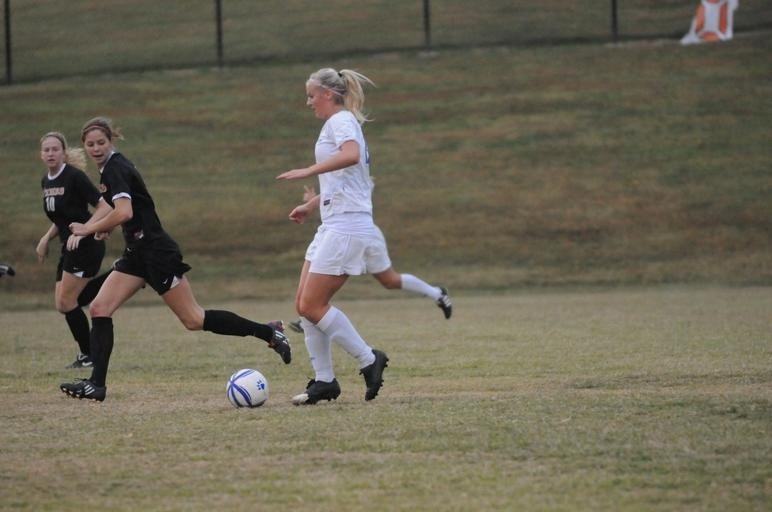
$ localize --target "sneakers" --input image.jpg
[360,349,390,401]
[437,287,452,320]
[61,378,107,403]
[0,264,15,277]
[267,320,293,365]
[65,353,94,370]
[288,320,305,334]
[292,378,342,407]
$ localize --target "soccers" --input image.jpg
[226,370,269,407]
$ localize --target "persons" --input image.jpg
[60,117,291,402]
[276,68,389,406]
[36,132,145,369]
[289,184,452,334]
